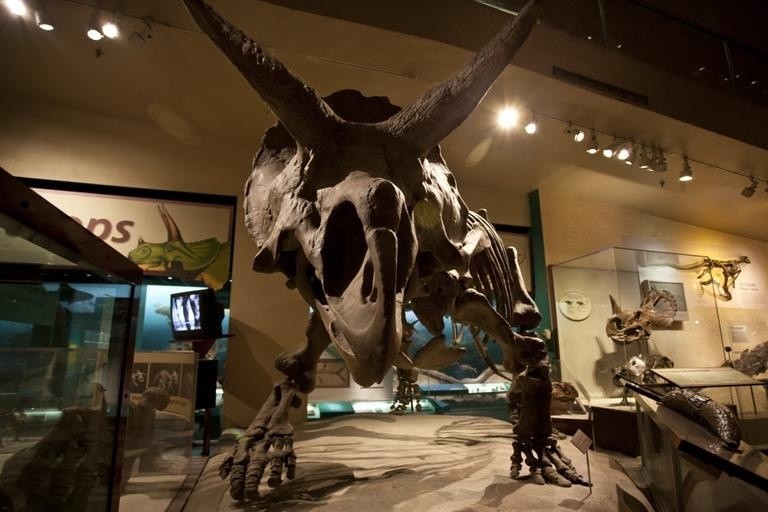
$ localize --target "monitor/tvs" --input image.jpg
[170,288,222,340]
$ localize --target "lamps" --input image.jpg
[740,178,760,198]
[563,123,694,184]
[34,3,155,54]
